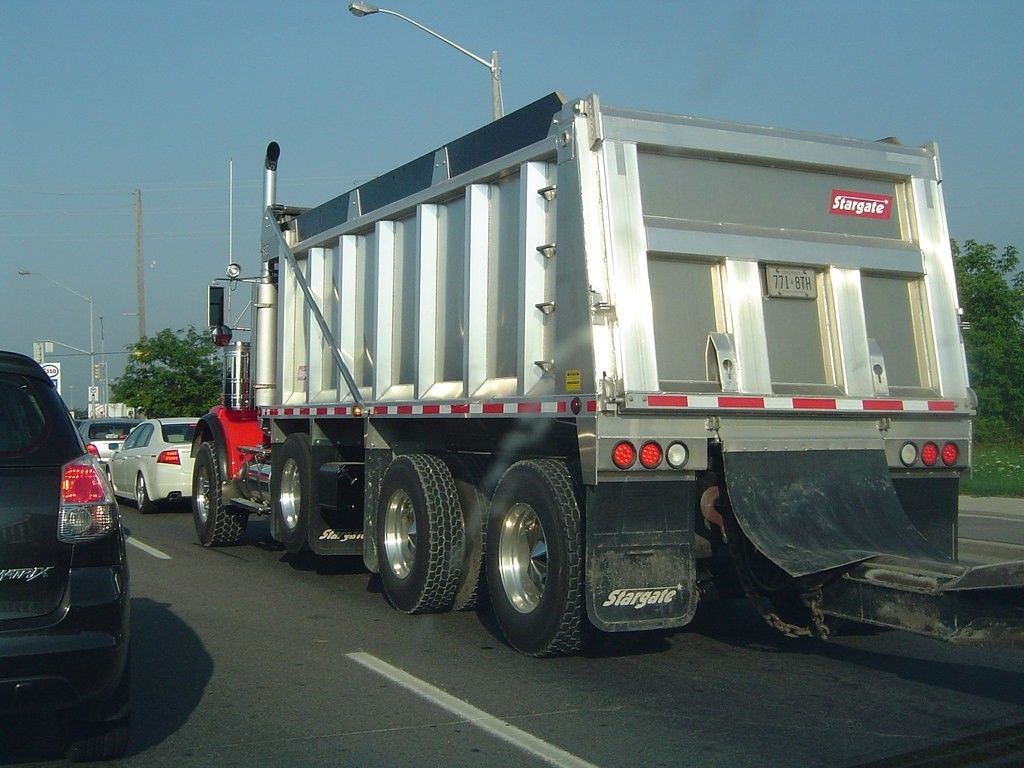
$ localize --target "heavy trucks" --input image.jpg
[190,91,1024,658]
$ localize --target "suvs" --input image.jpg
[0,351,130,762]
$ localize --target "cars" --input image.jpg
[74,418,141,462]
[106,417,205,515]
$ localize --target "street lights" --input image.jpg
[19,269,95,420]
